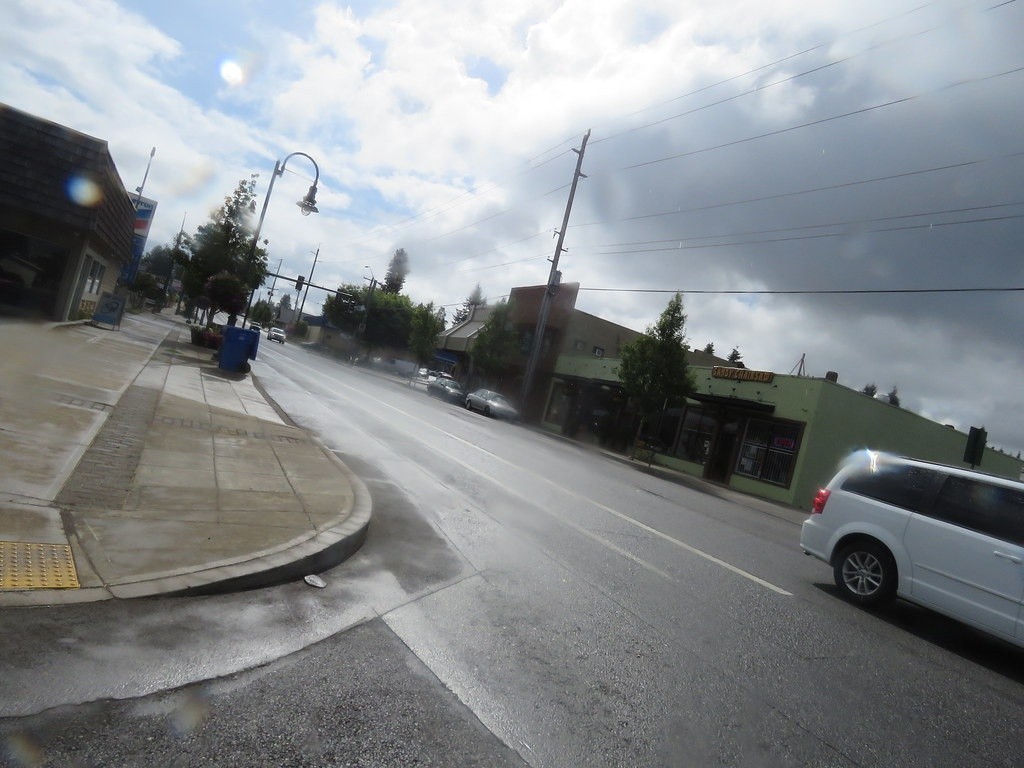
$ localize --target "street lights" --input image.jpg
[212,153,319,359]
[349,265,377,363]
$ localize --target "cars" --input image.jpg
[427,379,464,403]
[384,359,419,377]
[249,322,260,331]
[429,370,441,376]
[800,448,1024,651]
[464,389,518,418]
[267,327,286,344]
[419,368,428,376]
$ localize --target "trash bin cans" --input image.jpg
[218,325,260,374]
